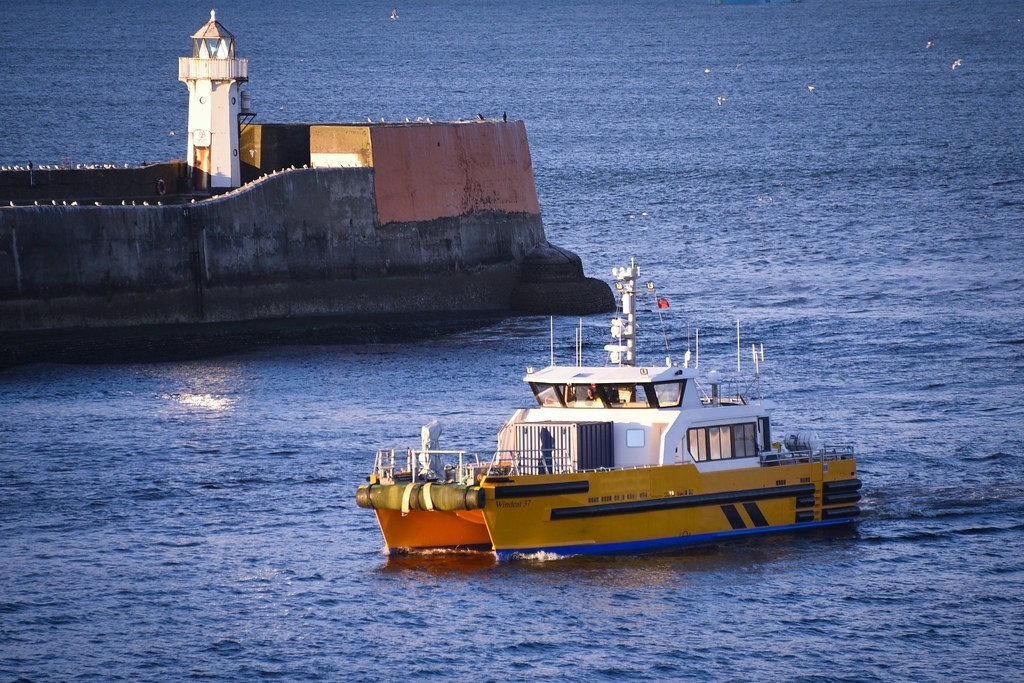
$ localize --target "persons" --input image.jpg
[586,388,597,401]
[502,112,507,122]
[142,160,146,165]
[29,161,33,171]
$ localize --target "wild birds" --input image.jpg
[689,35,963,107]
[2,106,512,217]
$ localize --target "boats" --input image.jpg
[354,252,862,560]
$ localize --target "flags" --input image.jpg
[657,298,670,308]
